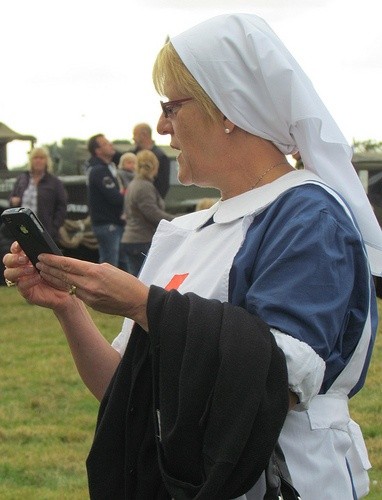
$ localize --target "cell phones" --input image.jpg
[2,208,63,275]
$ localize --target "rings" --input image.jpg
[69,285,77,294]
[5,279,20,287]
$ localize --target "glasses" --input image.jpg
[160,97,192,118]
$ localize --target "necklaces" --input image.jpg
[248,160,289,190]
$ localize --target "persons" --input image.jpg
[10,145,68,244]
[86,122,180,279]
[3,12,382,499]
